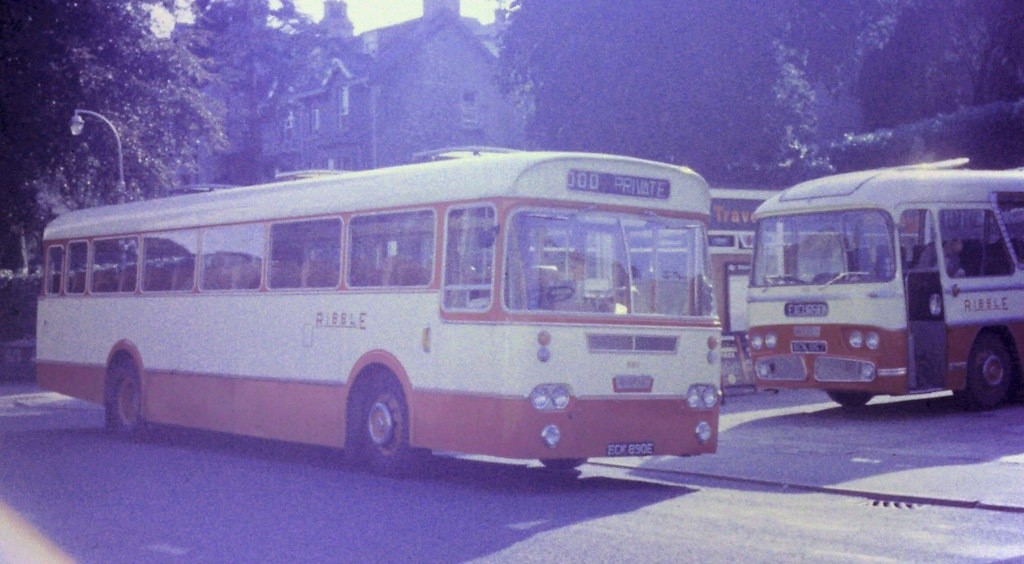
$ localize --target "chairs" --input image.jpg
[52,259,428,286]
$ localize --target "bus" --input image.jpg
[541,184,922,392]
[748,155,1024,411]
[35,145,722,478]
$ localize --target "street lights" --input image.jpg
[71,106,125,209]
